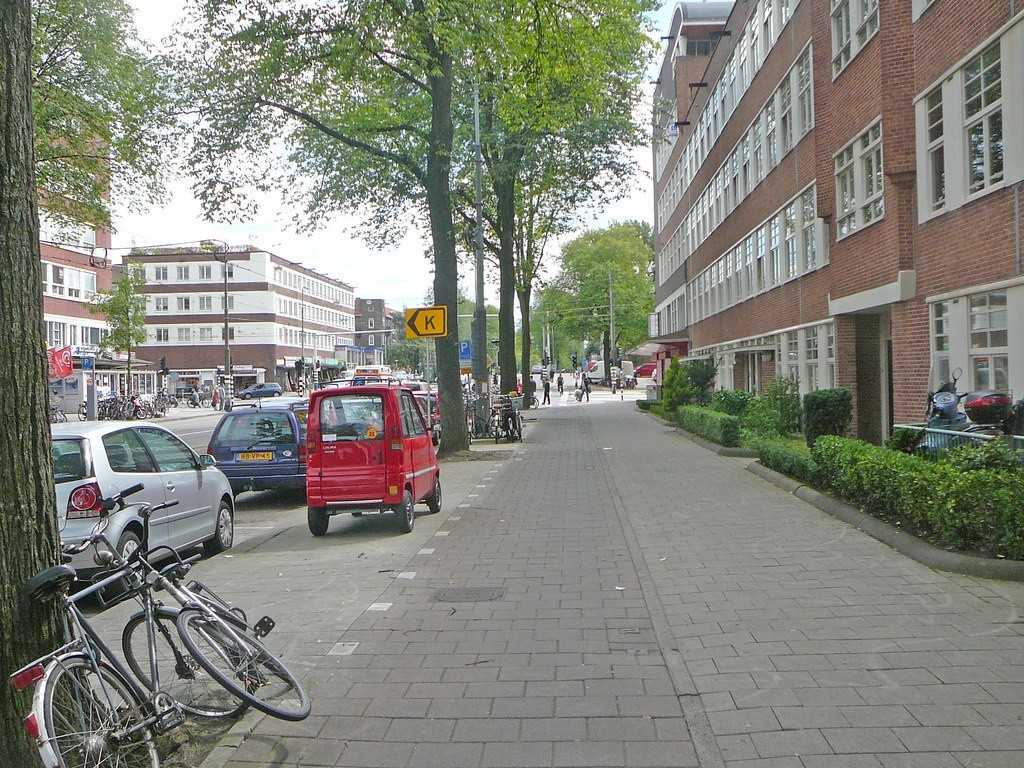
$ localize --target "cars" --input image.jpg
[237,382,283,399]
[206,408,306,502]
[52,419,234,600]
[318,363,446,420]
[304,385,445,535]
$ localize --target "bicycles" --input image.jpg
[186,390,212,408]
[50,381,179,422]
[557,379,564,395]
[93,498,312,722]
[8,483,257,768]
[462,382,523,444]
[529,397,540,409]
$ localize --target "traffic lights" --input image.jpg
[572,351,577,369]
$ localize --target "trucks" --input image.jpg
[585,360,634,384]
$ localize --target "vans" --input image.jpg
[633,363,657,377]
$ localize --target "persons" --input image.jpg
[517,379,522,389]
[556,374,564,395]
[530,375,537,396]
[578,372,590,402]
[550,368,555,383]
[212,389,220,405]
[190,389,201,407]
[541,379,551,405]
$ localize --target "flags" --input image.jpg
[51,346,74,379]
[45,348,59,380]
